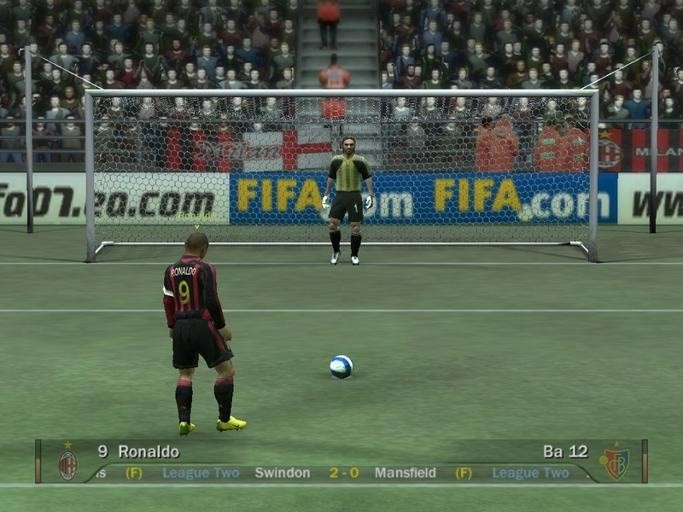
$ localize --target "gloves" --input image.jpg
[365,194,374,210]
[322,195,330,210]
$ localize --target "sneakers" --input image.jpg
[330,251,340,266]
[351,254,360,267]
[178,421,197,438]
[216,415,247,433]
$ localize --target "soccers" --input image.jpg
[330,355,353,378]
